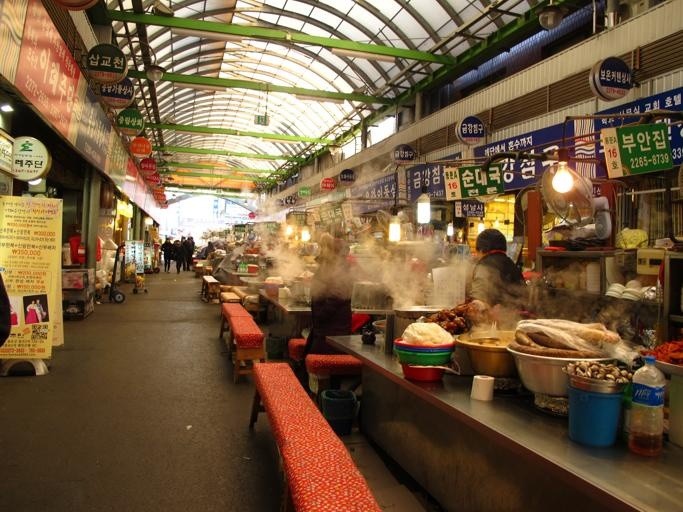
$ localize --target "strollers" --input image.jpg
[132,272,148,294]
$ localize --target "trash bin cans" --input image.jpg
[267,335,283,359]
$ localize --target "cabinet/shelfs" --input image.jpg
[536,249,682,373]
[62,266,96,319]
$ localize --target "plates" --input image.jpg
[604,279,657,301]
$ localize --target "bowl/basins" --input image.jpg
[240,248,260,274]
[394,304,455,320]
[398,360,453,382]
[287,275,312,306]
[561,366,632,395]
[456,329,518,377]
[263,276,285,298]
[393,337,457,365]
[372,319,385,336]
[505,342,619,396]
[586,261,600,294]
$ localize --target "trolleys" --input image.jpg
[99,243,127,304]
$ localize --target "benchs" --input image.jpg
[306,354,361,395]
[248,363,382,512]
[195,262,266,386]
[288,338,308,361]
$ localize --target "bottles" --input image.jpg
[630,356,666,459]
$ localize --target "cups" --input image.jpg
[362,326,376,344]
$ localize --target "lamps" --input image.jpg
[302,206,311,242]
[551,116,576,193]
[417,161,431,226]
[447,210,502,236]
[121,41,165,82]
[388,162,402,241]
[539,0,562,29]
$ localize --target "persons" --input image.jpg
[465,228,529,308]
[161,236,196,274]
[305,231,352,354]
[25,299,39,323]
[203,242,215,259]
[35,299,46,321]
